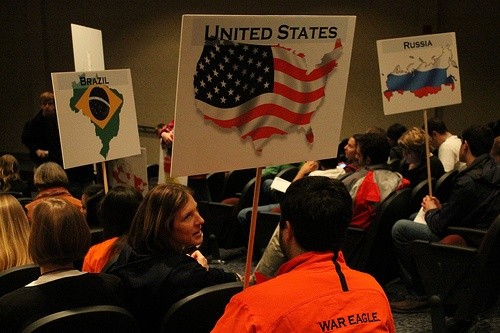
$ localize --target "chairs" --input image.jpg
[0,264,40,297]
[23,306,133,333]
[412,214,500,333]
[165,282,244,333]
[198,170,458,260]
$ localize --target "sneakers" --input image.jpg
[389,292,436,315]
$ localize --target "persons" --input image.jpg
[208,176,396,333]
[388,123,499,313]
[102,184,242,333]
[0,92,468,272]
[0,197,131,333]
